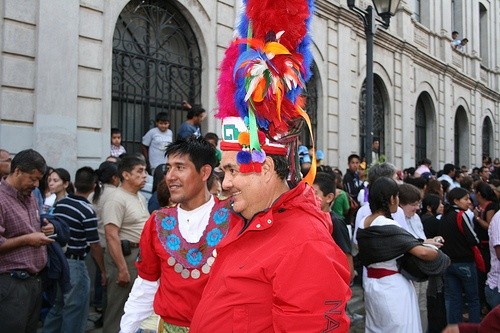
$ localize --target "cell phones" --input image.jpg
[47,233,58,239]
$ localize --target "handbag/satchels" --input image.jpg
[473,245,486,273]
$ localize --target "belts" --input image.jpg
[65,253,85,260]
[365,266,395,280]
[129,242,139,248]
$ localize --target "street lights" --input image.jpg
[346,0,402,171]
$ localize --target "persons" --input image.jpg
[0,148,178,333]
[451,31,459,40]
[452,38,469,51]
[204,131,222,165]
[176,99,206,140]
[117,134,245,333]
[342,137,500,333]
[294,137,354,254]
[141,112,173,175]
[110,126,126,157]
[189,0,352,333]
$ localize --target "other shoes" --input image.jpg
[93,302,104,312]
